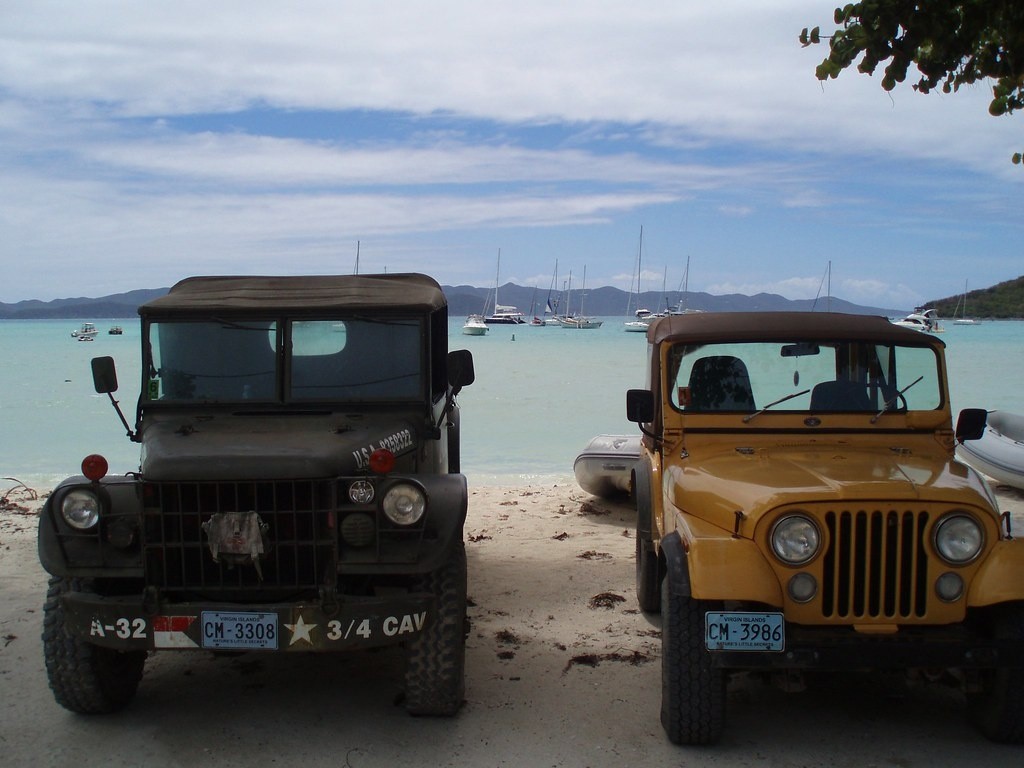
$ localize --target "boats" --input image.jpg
[71,321,123,342]
[953,409,1023,490]
[574,434,649,509]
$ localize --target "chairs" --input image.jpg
[683,355,757,410]
[810,380,875,411]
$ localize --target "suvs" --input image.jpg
[625,310,1023,747]
[36,271,475,718]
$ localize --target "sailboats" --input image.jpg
[622,224,709,332]
[889,276,984,336]
[462,246,605,335]
